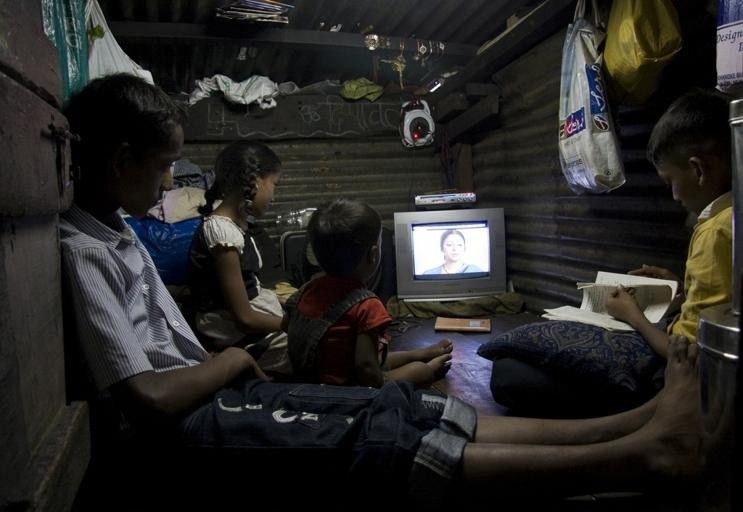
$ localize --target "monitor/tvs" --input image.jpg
[392,207,508,303]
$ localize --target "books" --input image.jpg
[214,0,295,25]
[435,315,490,334]
[541,269,682,332]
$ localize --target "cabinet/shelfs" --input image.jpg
[0,0,93,511]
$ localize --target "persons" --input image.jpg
[489,100,735,420]
[282,199,453,389]
[186,140,291,368]
[423,230,482,273]
[61,72,702,511]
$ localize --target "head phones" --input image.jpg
[397,100,437,150]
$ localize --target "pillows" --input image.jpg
[474,318,664,394]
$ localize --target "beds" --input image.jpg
[375,308,694,512]
